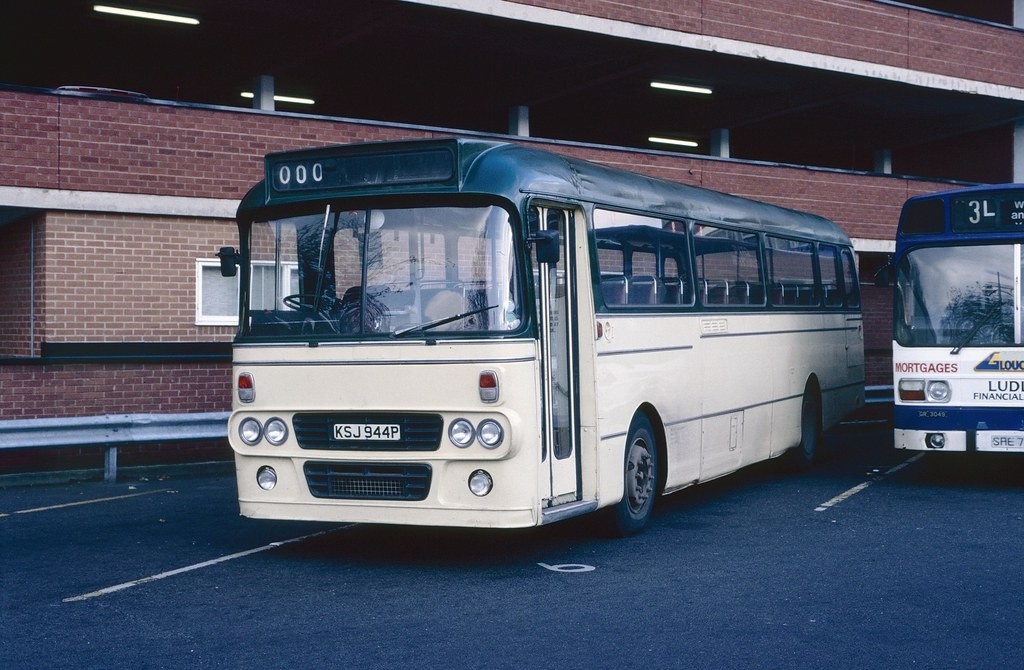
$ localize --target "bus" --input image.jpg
[892,183,1024,451]
[216,138,864,537]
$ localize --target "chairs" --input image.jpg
[334,276,838,332]
